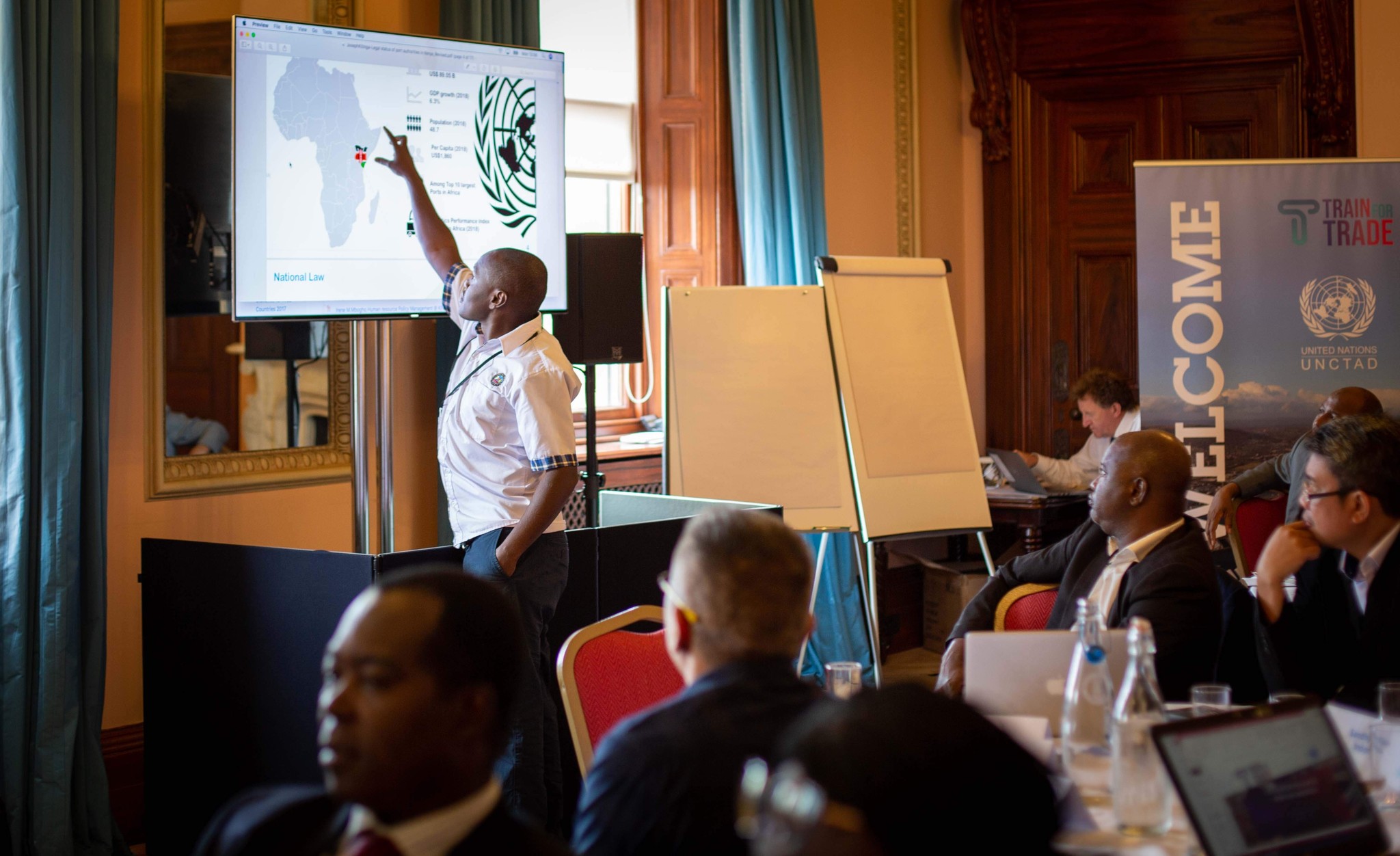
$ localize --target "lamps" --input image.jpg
[548,233,643,526]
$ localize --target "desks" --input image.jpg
[945,487,1097,558]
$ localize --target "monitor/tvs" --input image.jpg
[160,70,232,317]
[230,13,565,320]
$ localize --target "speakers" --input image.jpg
[245,319,327,359]
[552,233,645,365]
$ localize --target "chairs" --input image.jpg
[995,581,1060,634]
[1224,480,1304,585]
[558,597,704,778]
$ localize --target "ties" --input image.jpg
[340,829,403,856]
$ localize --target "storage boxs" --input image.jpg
[891,548,1000,654]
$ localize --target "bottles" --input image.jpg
[1107,617,1177,838]
[1060,599,1118,790]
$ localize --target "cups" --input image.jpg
[1378,682,1400,725]
[1189,685,1231,719]
[1365,722,1399,813]
[825,661,862,701]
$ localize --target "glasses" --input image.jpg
[739,760,860,840]
[1298,487,1373,503]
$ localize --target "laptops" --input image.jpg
[1150,697,1400,856]
[964,632,1135,740]
[983,446,1093,496]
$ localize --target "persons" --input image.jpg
[165,401,229,459]
[196,566,576,856]
[933,369,1400,718]
[574,507,1075,856]
[375,125,582,844]
